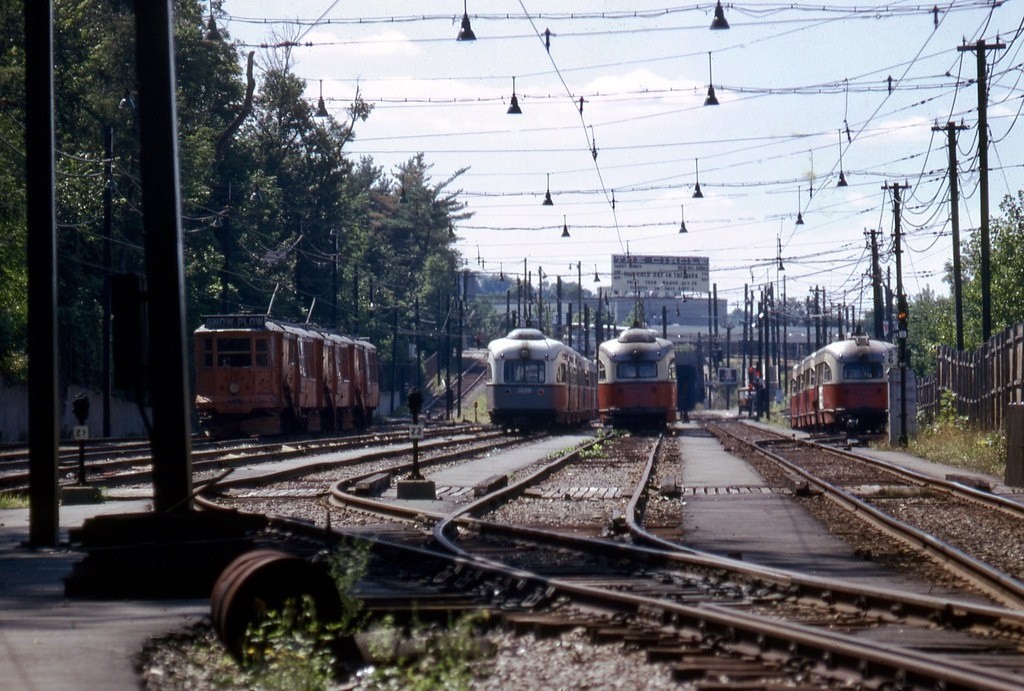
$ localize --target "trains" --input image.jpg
[194,311,380,436]
[789,331,897,434]
[486,327,599,432]
[594,329,679,425]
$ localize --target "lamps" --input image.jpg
[691,158,703,198]
[675,301,680,316]
[593,263,600,282]
[506,77,522,114]
[704,52,719,105]
[679,205,687,233]
[561,215,570,237]
[313,80,329,117]
[795,186,804,224]
[710,0,730,29]
[682,264,688,279]
[456,0,476,41]
[543,173,554,205]
[836,129,848,186]
[498,263,504,282]
[777,239,784,270]
[682,290,686,301]
